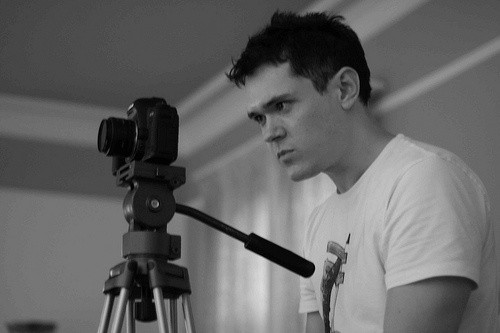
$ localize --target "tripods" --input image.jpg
[97,162,196,333]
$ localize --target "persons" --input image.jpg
[223,6,500,332]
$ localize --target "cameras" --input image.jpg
[98,97,178,175]
